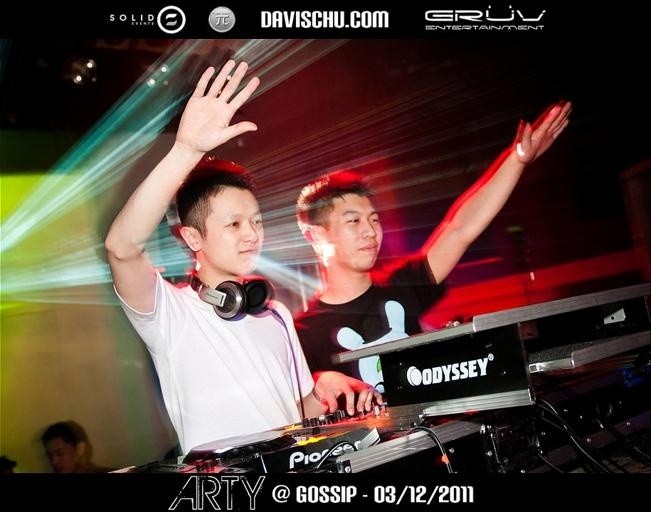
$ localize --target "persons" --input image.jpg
[32,418,119,477]
[285,96,575,404]
[97,55,389,454]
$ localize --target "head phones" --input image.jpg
[191,275,275,321]
